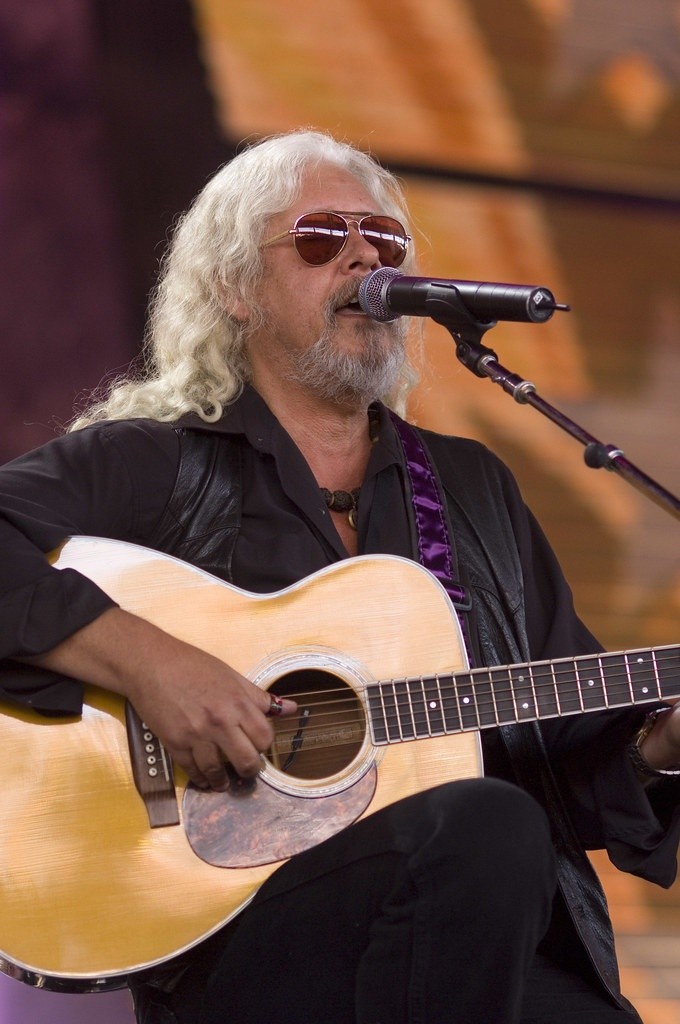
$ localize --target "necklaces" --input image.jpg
[321,486,363,529]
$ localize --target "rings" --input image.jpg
[265,693,282,716]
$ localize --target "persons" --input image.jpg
[0,132,680,1024]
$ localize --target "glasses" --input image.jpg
[257,211,412,269]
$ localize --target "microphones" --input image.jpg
[358,266,571,324]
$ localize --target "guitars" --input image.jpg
[3,529,680,999]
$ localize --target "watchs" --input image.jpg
[624,703,680,779]
